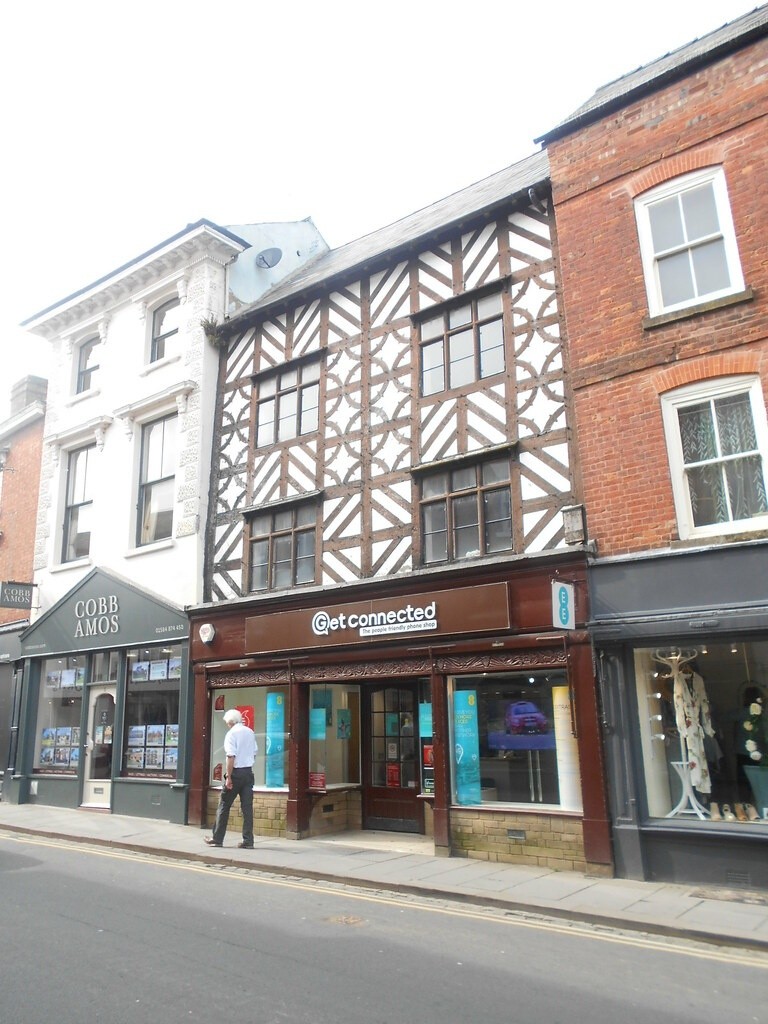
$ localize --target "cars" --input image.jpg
[505,701,548,736]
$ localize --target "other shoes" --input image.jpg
[710,802,722,820]
[746,803,760,821]
[238,843,253,848]
[723,805,735,819]
[735,804,747,820]
[204,835,222,847]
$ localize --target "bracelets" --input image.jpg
[226,774,229,777]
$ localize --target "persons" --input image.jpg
[204,709,257,849]
